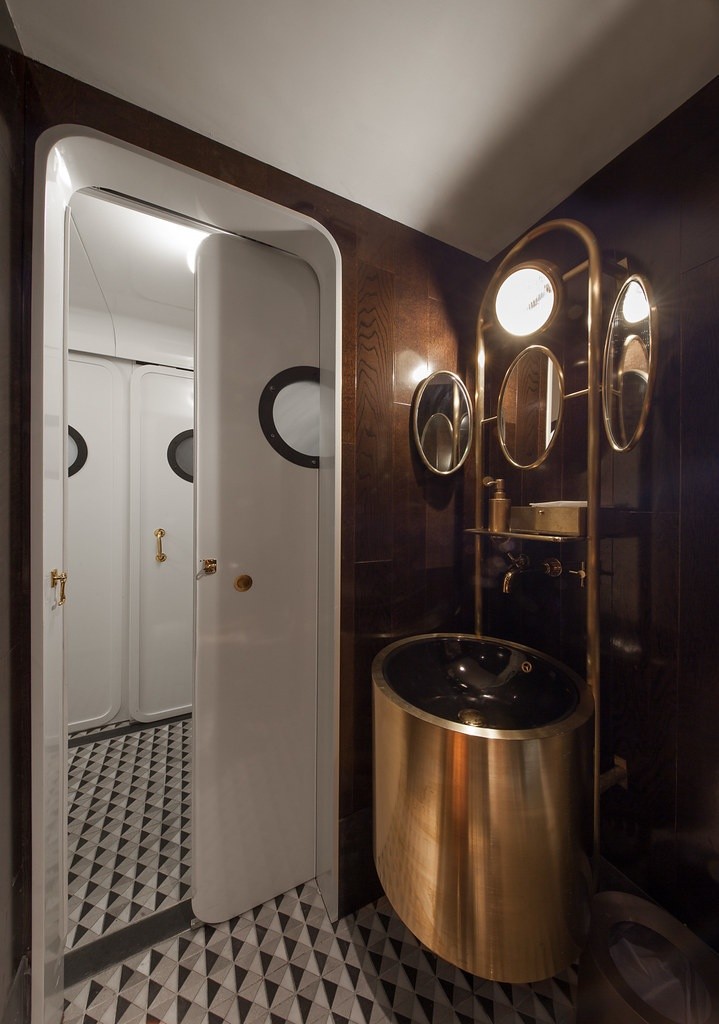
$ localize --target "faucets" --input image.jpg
[499,550,534,598]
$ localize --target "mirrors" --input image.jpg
[498,345,565,471]
[612,334,649,450]
[601,274,658,455]
[422,414,470,473]
[413,371,474,476]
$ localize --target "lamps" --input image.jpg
[492,258,566,341]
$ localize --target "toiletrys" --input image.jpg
[483,478,511,534]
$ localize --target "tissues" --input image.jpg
[508,499,628,540]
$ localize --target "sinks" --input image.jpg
[366,629,594,987]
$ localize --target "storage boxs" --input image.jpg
[511,505,617,537]
